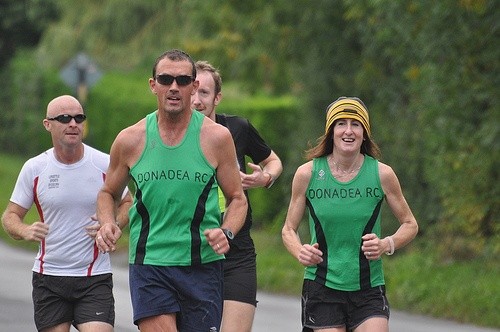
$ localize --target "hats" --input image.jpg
[326,97,370,138]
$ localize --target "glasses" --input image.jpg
[153,74,195,86]
[47,115,87,124]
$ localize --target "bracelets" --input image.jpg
[384,237,394,256]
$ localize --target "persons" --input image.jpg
[189,59,282,332]
[1,95,134,332]
[281,96,418,332]
[96,48,248,332]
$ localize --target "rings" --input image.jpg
[217,244,221,249]
[368,252,371,256]
[97,236,102,239]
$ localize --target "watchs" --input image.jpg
[265,172,275,189]
[219,227,234,246]
